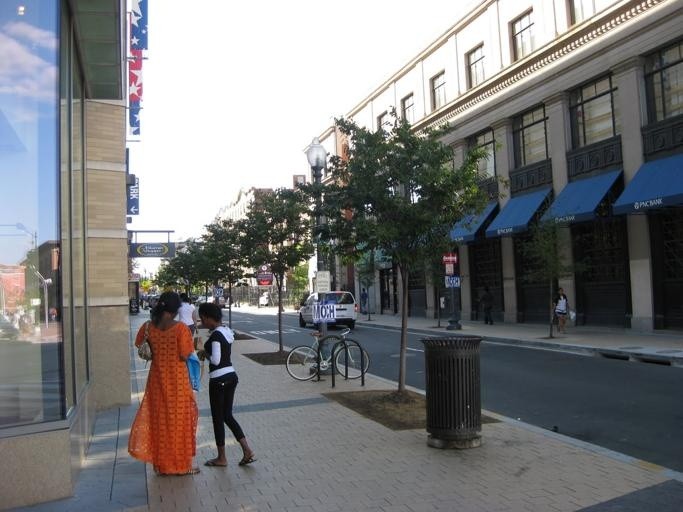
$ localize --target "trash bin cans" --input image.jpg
[416,335,485,449]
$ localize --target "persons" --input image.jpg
[4,310,34,339]
[177,292,198,353]
[360,288,368,314]
[140,295,159,308]
[480,286,494,324]
[188,299,196,310]
[552,288,570,334]
[127,291,201,477]
[50,305,57,321]
[199,303,258,466]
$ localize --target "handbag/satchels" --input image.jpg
[138,321,153,363]
[569,311,576,321]
[194,332,203,350]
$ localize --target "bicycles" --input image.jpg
[286,327,370,381]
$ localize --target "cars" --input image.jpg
[260,292,280,306]
[188,294,226,308]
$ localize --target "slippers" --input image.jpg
[239,456,256,466]
[204,458,227,468]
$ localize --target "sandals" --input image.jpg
[178,468,200,475]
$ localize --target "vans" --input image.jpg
[297,291,360,330]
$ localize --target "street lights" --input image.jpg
[305,138,330,271]
